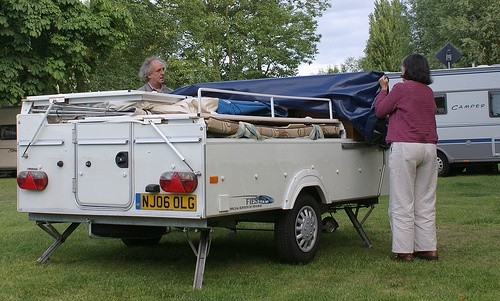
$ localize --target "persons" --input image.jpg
[136,57,173,94]
[374,52,441,261]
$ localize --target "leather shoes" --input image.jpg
[413,250,438,261]
[391,252,412,261]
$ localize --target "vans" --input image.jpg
[370,64,500,177]
